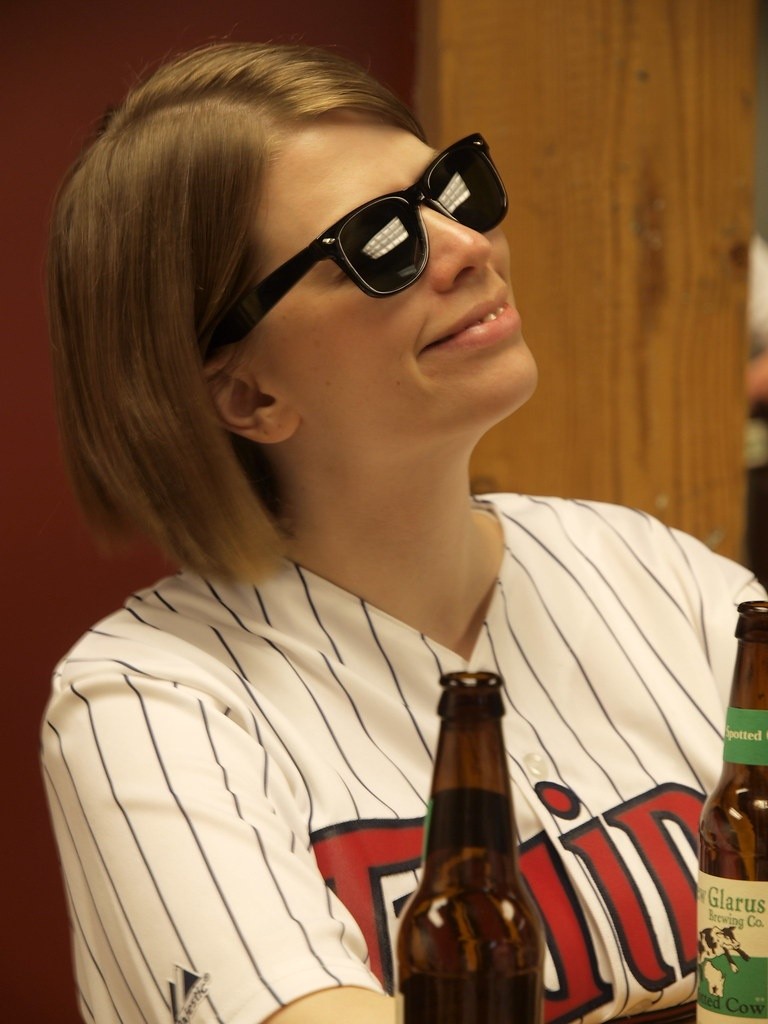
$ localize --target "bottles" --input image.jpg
[695,600,768,1024]
[394,672,545,1024]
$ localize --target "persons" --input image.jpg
[44,47,767,1021]
[742,234,768,588]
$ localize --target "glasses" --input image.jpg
[205,134,510,363]
